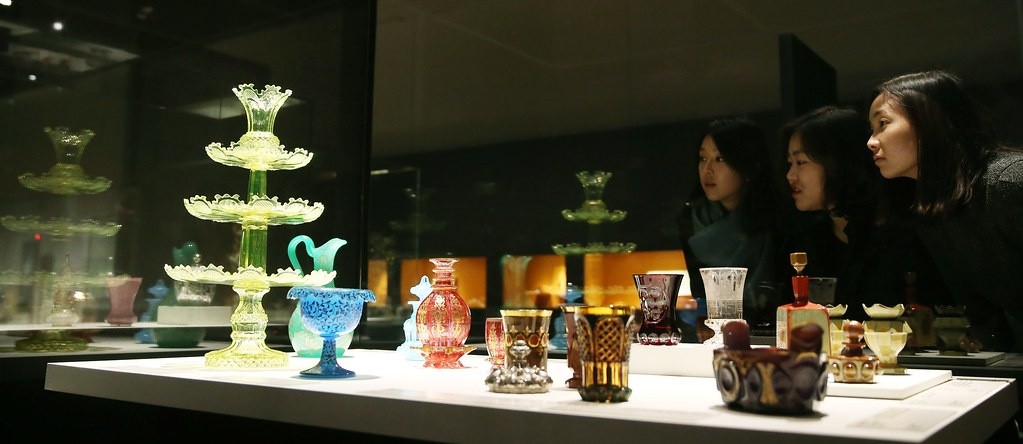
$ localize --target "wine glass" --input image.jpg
[287,286,377,377]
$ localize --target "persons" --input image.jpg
[867,71,1023,352]
[678,117,816,320]
[781,105,908,322]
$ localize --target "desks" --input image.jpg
[43,348,1020,444]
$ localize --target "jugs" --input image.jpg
[288,235,354,358]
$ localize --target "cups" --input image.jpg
[485,318,508,365]
[104,276,143,326]
[633,274,685,346]
[700,267,748,345]
[499,309,553,373]
[573,305,636,402]
[561,306,596,388]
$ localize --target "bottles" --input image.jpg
[416,257,477,369]
[776,253,832,357]
